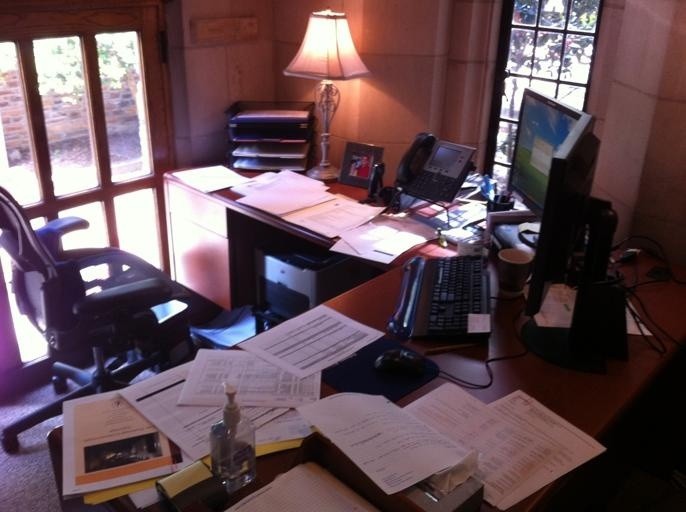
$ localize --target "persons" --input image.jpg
[346,159,358,176]
[355,154,370,179]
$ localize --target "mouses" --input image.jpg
[374,348,429,376]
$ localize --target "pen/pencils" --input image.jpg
[493,183,512,203]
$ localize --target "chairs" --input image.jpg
[0,185,196,456]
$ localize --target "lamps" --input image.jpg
[280,8,371,181]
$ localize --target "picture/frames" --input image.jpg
[338,140,385,189]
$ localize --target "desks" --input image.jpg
[161,157,479,328]
[44,251,686,511]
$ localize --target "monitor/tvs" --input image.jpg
[519,114,620,368]
[507,88,595,248]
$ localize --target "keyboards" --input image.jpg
[412,255,492,344]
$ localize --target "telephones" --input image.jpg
[394,132,478,204]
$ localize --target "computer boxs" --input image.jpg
[252,234,354,320]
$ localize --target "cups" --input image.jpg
[488,195,514,215]
[496,247,536,300]
